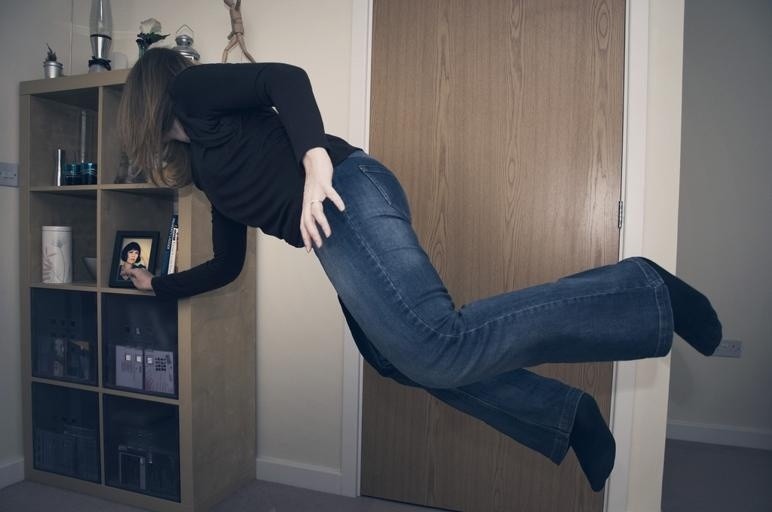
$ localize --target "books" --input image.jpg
[161,214,178,276]
[167,225,176,275]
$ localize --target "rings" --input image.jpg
[310,197,323,206]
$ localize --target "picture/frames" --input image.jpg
[108,229,159,290]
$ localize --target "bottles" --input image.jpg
[40,224,73,284]
[51,148,67,186]
[88,1,113,73]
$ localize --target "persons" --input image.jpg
[119,241,145,280]
[113,47,724,491]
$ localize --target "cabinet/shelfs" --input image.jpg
[17,66,258,510]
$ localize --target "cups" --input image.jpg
[64,162,81,186]
[79,162,97,184]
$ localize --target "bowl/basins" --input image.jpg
[81,255,97,279]
